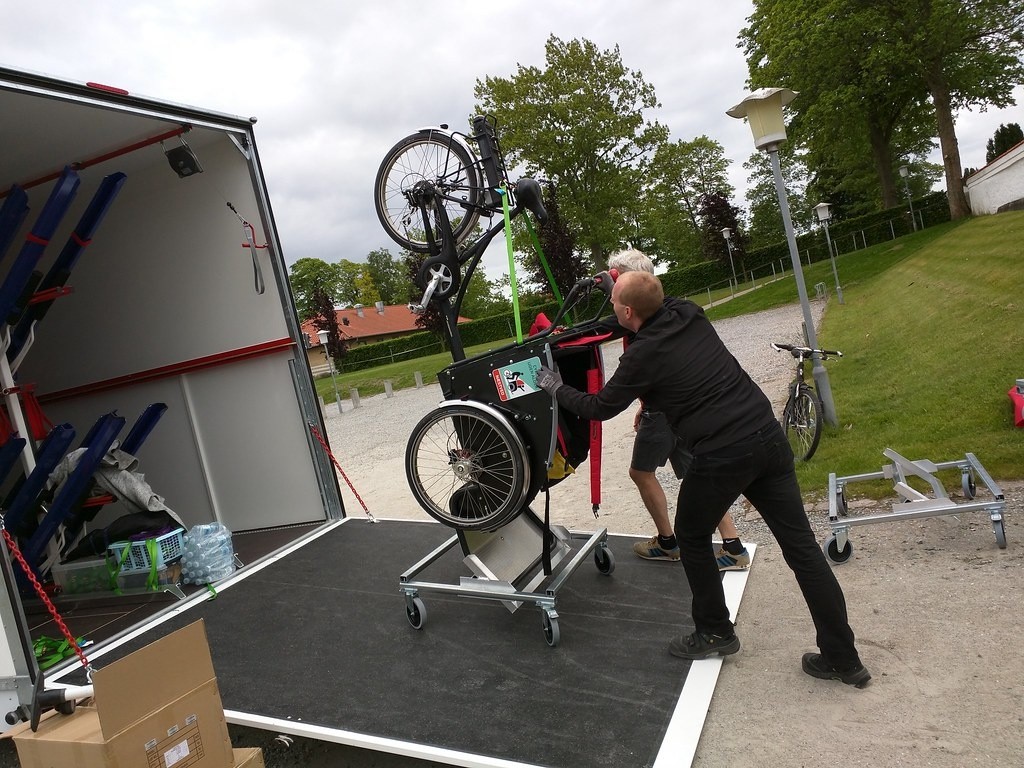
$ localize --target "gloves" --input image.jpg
[592,270,614,293]
[536,360,564,396]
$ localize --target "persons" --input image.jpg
[607,249,751,571]
[536,270,872,685]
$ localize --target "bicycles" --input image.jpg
[372,107,627,532]
[769,341,844,464]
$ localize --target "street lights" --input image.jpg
[726,87,840,430]
[720,227,739,291]
[316,329,344,415]
[811,201,845,305]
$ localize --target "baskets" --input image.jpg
[107,527,185,576]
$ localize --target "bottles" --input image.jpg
[180,522,236,585]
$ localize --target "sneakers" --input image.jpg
[632,536,681,562]
[801,652,872,689]
[669,631,739,660]
[714,548,752,572]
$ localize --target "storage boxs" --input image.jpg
[0,618,237,768]
[232,747,266,768]
[50,553,119,594]
[112,560,182,589]
